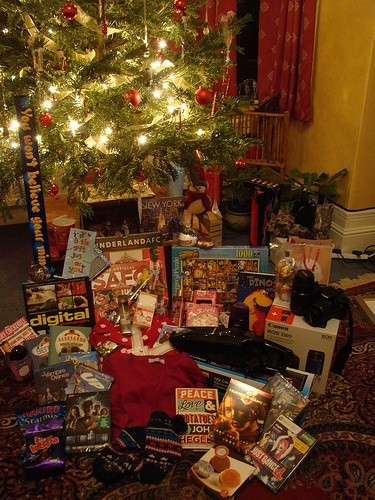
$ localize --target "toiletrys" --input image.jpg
[171,290,185,327]
[8,345,32,381]
[228,302,249,329]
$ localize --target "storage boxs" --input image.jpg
[269,235,306,265]
[264,284,340,394]
[203,211,222,246]
[185,289,220,327]
[133,291,157,328]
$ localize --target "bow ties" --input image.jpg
[84,416,91,420]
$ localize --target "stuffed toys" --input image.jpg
[184,184,212,214]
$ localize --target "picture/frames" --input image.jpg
[352,296,375,328]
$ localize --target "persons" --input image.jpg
[100,407,108,417]
[103,220,112,237]
[1,340,11,352]
[66,406,80,428]
[214,392,260,443]
[147,247,167,303]
[42,387,58,403]
[92,405,100,416]
[122,220,129,235]
[75,400,99,430]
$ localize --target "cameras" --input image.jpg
[304,284,352,330]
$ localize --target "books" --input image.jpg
[0,272,317,494]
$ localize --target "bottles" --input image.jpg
[278,250,296,302]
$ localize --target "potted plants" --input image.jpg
[292,167,347,240]
[208,124,265,230]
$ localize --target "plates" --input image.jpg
[196,239,215,248]
[190,448,256,498]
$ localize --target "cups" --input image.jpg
[52,217,76,252]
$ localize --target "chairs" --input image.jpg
[230,111,289,179]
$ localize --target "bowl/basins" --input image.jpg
[178,236,197,246]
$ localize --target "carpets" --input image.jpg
[1,272,375,500]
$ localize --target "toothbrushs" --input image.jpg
[126,268,149,295]
[130,270,153,300]
[152,275,159,290]
